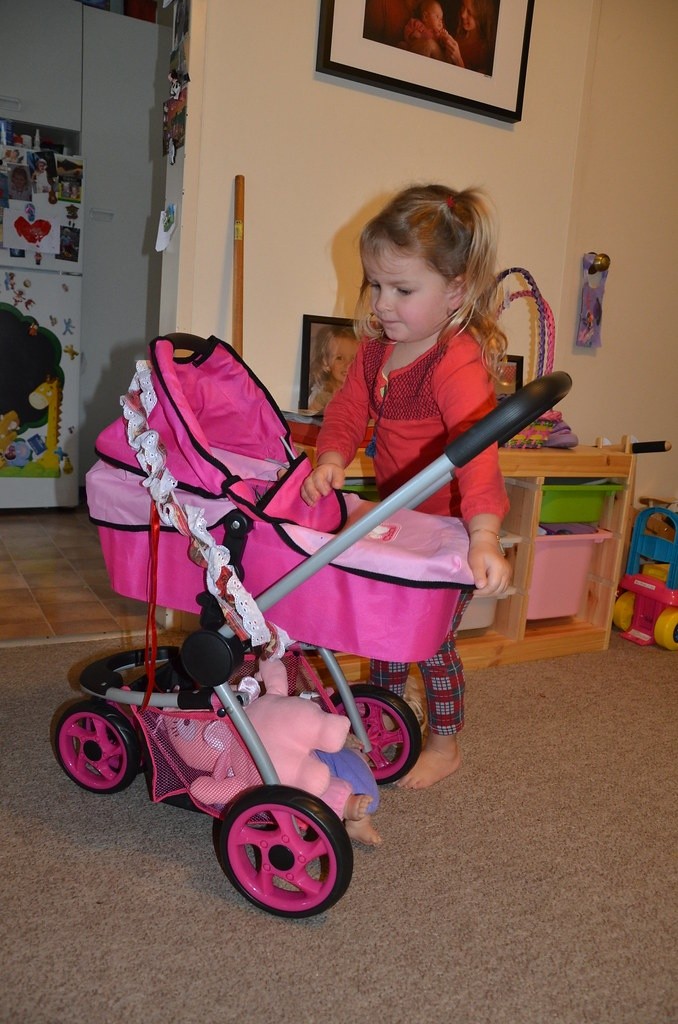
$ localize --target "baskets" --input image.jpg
[483,267,562,448]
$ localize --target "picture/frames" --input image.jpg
[491,353,523,398]
[300,314,368,418]
[315,0,534,124]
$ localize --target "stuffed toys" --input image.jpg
[163,653,352,803]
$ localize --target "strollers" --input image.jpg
[51,329,571,921]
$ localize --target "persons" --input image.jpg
[321,778,383,846]
[32,159,51,193]
[308,328,369,410]
[70,186,80,199]
[365,0,496,74]
[61,228,78,255]
[9,167,32,201]
[299,184,516,791]
[0,149,19,170]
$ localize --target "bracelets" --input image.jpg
[470,529,501,542]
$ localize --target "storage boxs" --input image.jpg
[527,528,614,621]
[538,484,627,524]
[458,585,517,631]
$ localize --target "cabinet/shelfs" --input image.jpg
[281,444,637,690]
